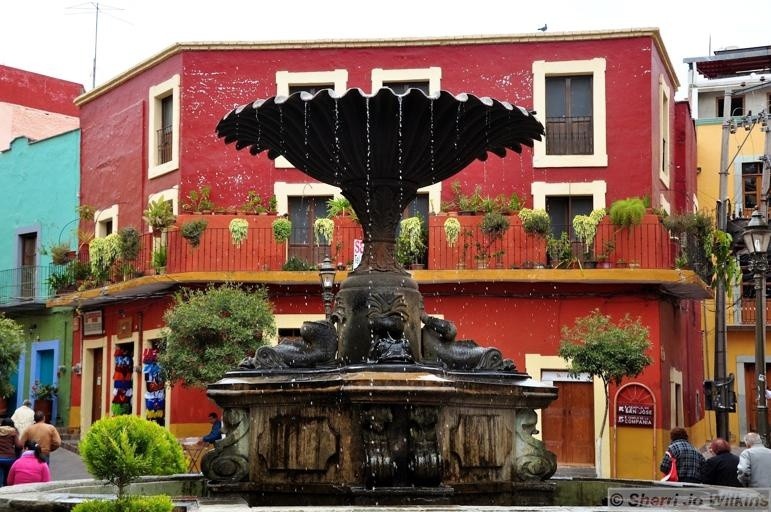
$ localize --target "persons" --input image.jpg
[7,438,53,485]
[735,432,771,488]
[9,399,36,440]
[19,409,61,467]
[660,426,707,485]
[1,416,22,487]
[698,437,749,488]
[197,412,222,450]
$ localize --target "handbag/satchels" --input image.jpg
[660,449,681,482]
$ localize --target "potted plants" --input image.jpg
[324,197,352,219]
[0,314,25,417]
[399,217,426,269]
[436,181,524,216]
[30,379,58,425]
[140,195,176,277]
[185,188,278,218]
[54,225,140,294]
[456,230,628,271]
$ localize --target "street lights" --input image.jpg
[318,252,337,321]
[741,204,770,441]
